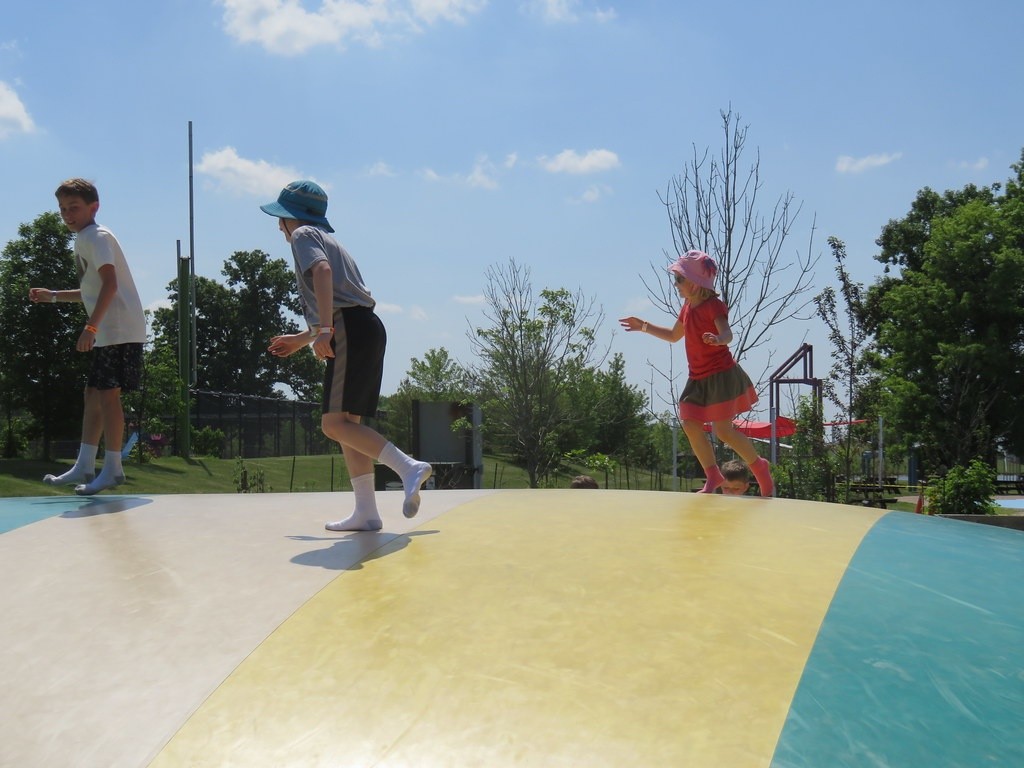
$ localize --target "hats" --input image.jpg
[665,250,717,290]
[260,180,335,233]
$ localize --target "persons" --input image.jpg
[260,180,433,532]
[27,176,145,497]
[619,250,775,498]
[719,459,749,496]
[571,475,600,489]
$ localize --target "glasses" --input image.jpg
[674,275,686,284]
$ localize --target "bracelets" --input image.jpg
[83,325,98,336]
[641,321,649,332]
[318,327,335,336]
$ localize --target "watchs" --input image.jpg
[52,290,57,303]
